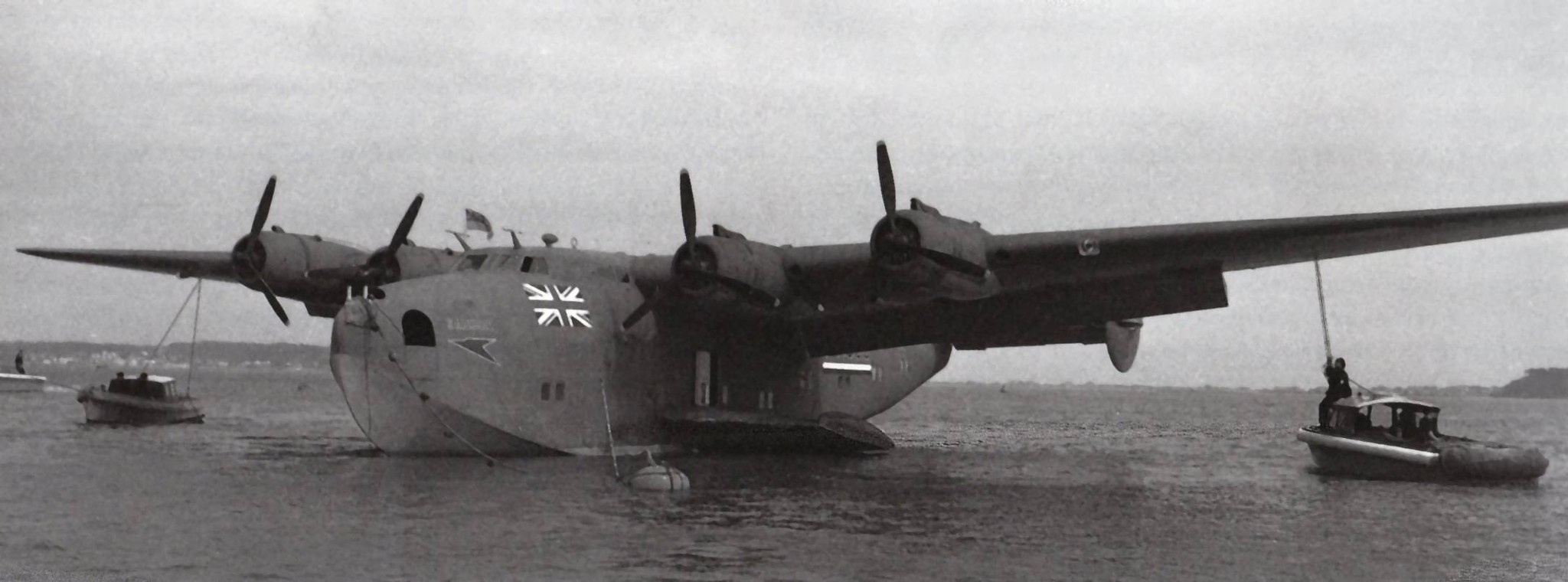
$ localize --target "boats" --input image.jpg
[77,375,207,427]
[1297,393,1548,485]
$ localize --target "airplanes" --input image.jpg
[13,139,1567,459]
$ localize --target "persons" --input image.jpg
[13,349,26,376]
[139,372,149,380]
[1317,356,1352,430]
[116,371,124,379]
